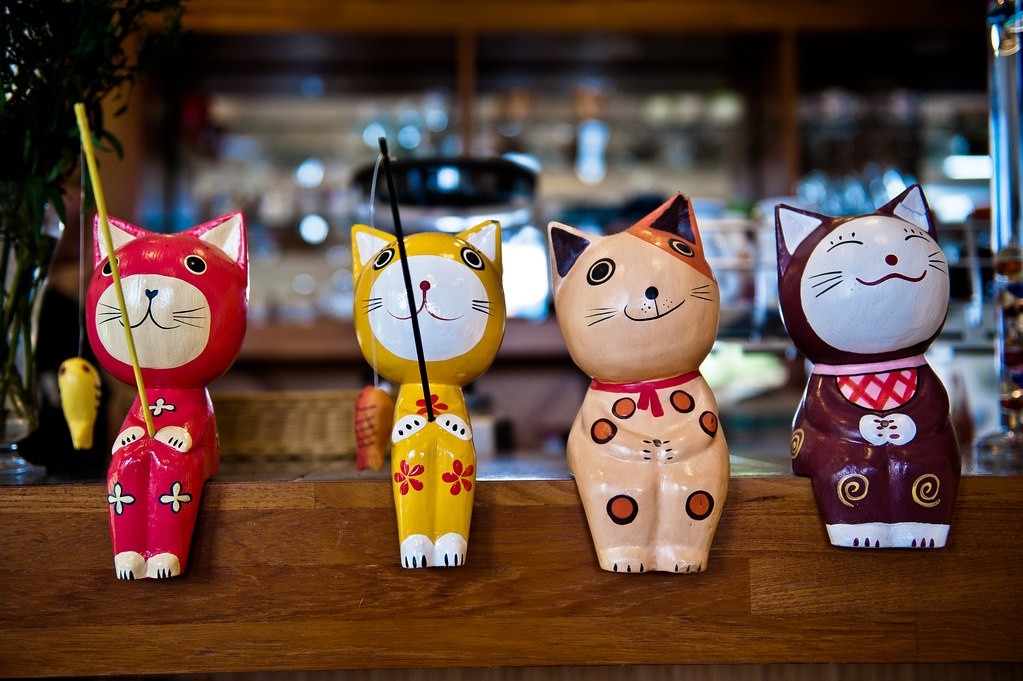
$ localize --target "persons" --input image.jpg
[30,276,108,485]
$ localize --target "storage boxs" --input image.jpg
[210,389,359,457]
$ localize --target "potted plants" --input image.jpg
[0,0,189,487]
